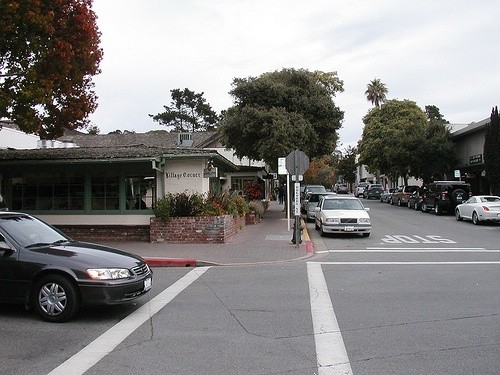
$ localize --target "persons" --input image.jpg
[274,185,285,204]
[134,194,147,209]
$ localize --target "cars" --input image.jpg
[380,188,397,203]
[392,185,424,209]
[455,196,500,225]
[300,185,372,237]
[1,211,153,324]
[335,184,349,193]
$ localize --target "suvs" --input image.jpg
[423,182,469,216]
[354,183,384,199]
